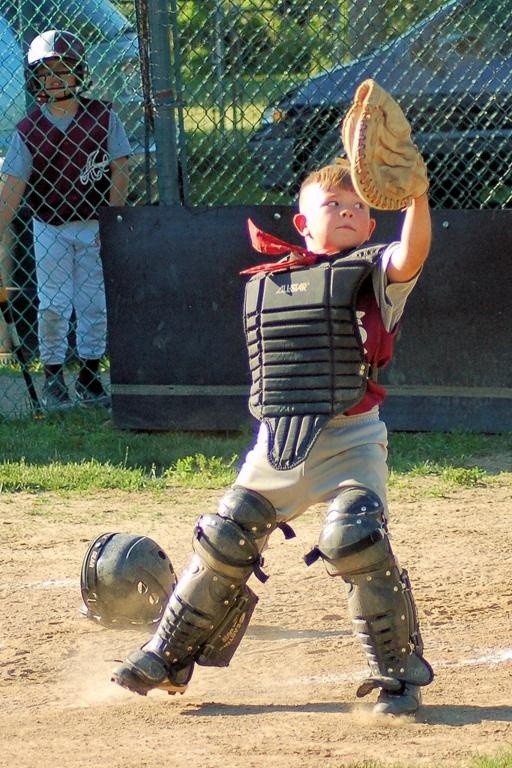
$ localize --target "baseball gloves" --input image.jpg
[341,79,430,212]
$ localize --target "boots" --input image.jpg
[44,365,69,400]
[114,650,189,694]
[374,682,421,715]
[76,375,106,399]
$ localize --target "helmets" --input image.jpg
[80,531,175,629]
[28,30,85,64]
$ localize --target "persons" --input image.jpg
[0,29,130,410]
[112,78,433,715]
